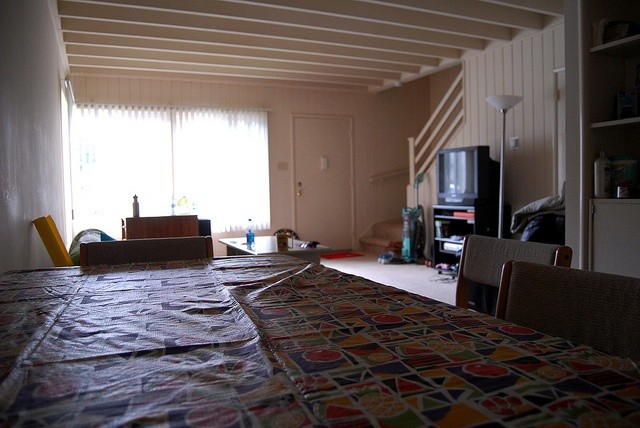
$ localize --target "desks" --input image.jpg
[220,235,333,264]
[0,263,640,428]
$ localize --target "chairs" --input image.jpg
[80,236,214,266]
[125,215,200,239]
[494,258,640,363]
[274,228,299,240]
[455,234,573,321]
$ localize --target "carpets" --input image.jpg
[320,250,365,260]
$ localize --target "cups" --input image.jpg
[276,233,293,251]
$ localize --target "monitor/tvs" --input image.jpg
[434,144,500,207]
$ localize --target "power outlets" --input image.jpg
[509,136,520,150]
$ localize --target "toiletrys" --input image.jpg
[594,150,611,198]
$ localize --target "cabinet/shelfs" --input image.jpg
[580,1,640,280]
[433,204,513,277]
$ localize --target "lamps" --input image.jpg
[485,95,523,237]
